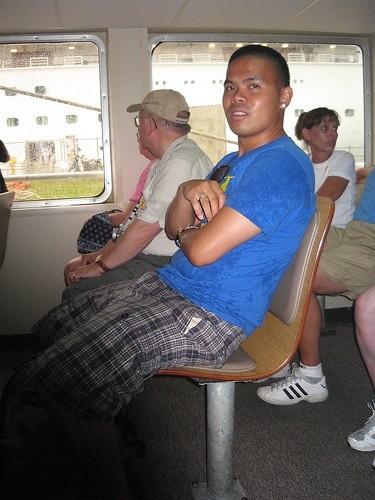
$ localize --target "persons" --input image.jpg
[251,164,375,405]
[63,133,156,284]
[346,285,375,468]
[64,89,214,302]
[296,108,355,228]
[0,45,316,500]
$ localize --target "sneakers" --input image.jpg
[257,362,327,405]
[347,398,375,452]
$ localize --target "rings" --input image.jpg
[200,195,208,199]
[72,272,75,275]
[70,269,73,272]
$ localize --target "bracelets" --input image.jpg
[175,225,198,247]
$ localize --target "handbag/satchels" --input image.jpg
[77,209,123,255]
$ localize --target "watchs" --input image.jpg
[94,254,109,272]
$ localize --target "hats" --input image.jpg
[127,88,190,124]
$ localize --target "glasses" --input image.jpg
[134,115,158,130]
[208,165,230,183]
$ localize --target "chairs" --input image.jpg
[155,196,335,500]
